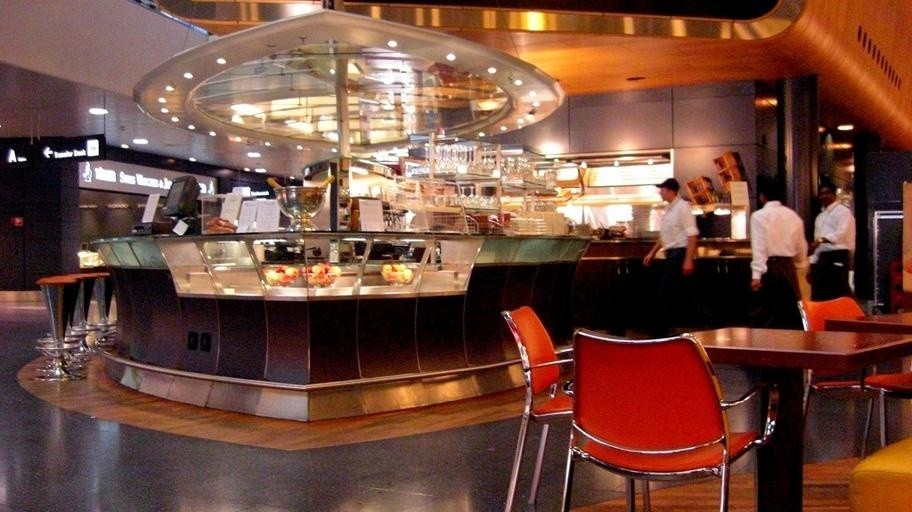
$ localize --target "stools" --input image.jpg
[848,439,912,512]
[33,272,116,382]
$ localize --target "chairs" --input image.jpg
[561,328,780,512]
[501,304,636,512]
[864,372,912,452]
[797,296,912,459]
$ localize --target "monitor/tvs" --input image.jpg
[157,176,200,219]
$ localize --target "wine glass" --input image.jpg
[460,184,499,209]
[483,155,528,174]
[423,144,477,174]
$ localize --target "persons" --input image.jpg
[642,177,701,327]
[807,178,856,302]
[748,177,810,389]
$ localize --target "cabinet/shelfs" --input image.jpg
[476,144,547,235]
[410,132,500,236]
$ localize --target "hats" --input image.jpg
[656,179,679,191]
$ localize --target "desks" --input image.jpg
[825,312,912,444]
[690,327,911,512]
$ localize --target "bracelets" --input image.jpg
[817,239,823,244]
